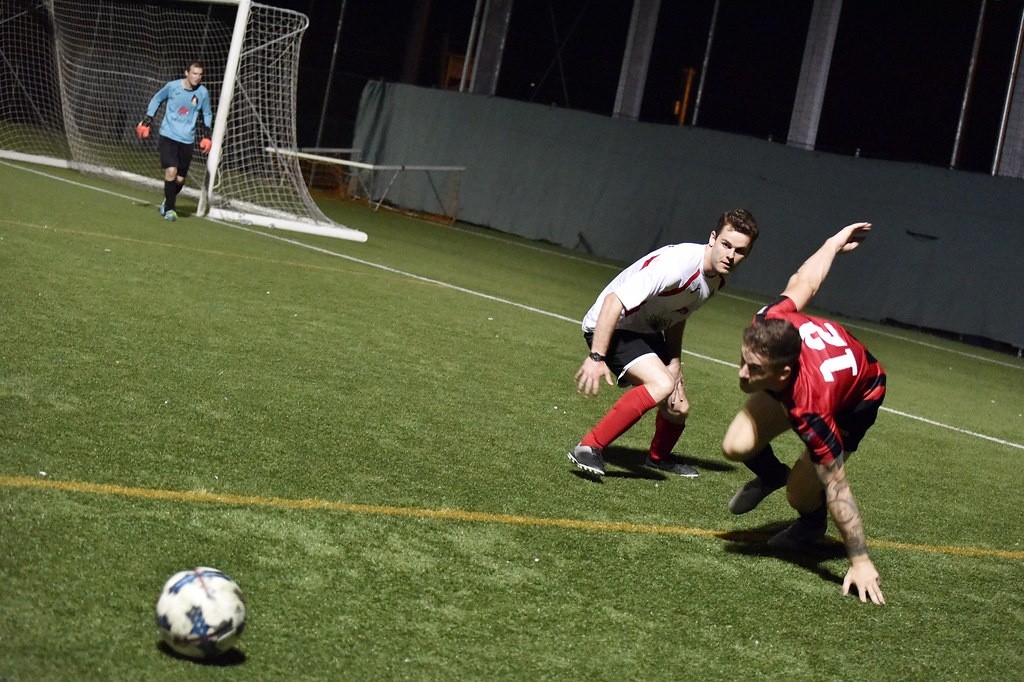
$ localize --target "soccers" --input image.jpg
[156,565,248,660]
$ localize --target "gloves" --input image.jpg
[200,130,211,154]
[137,116,153,139]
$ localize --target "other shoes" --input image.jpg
[160,203,165,215]
[164,210,178,221]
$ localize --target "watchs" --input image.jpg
[589,353,605,361]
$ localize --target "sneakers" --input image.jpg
[728,462,792,514]
[645,452,698,477]
[567,442,606,475]
[767,518,827,547]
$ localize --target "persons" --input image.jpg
[136,62,213,223]
[722,221,886,606]
[567,209,760,478]
